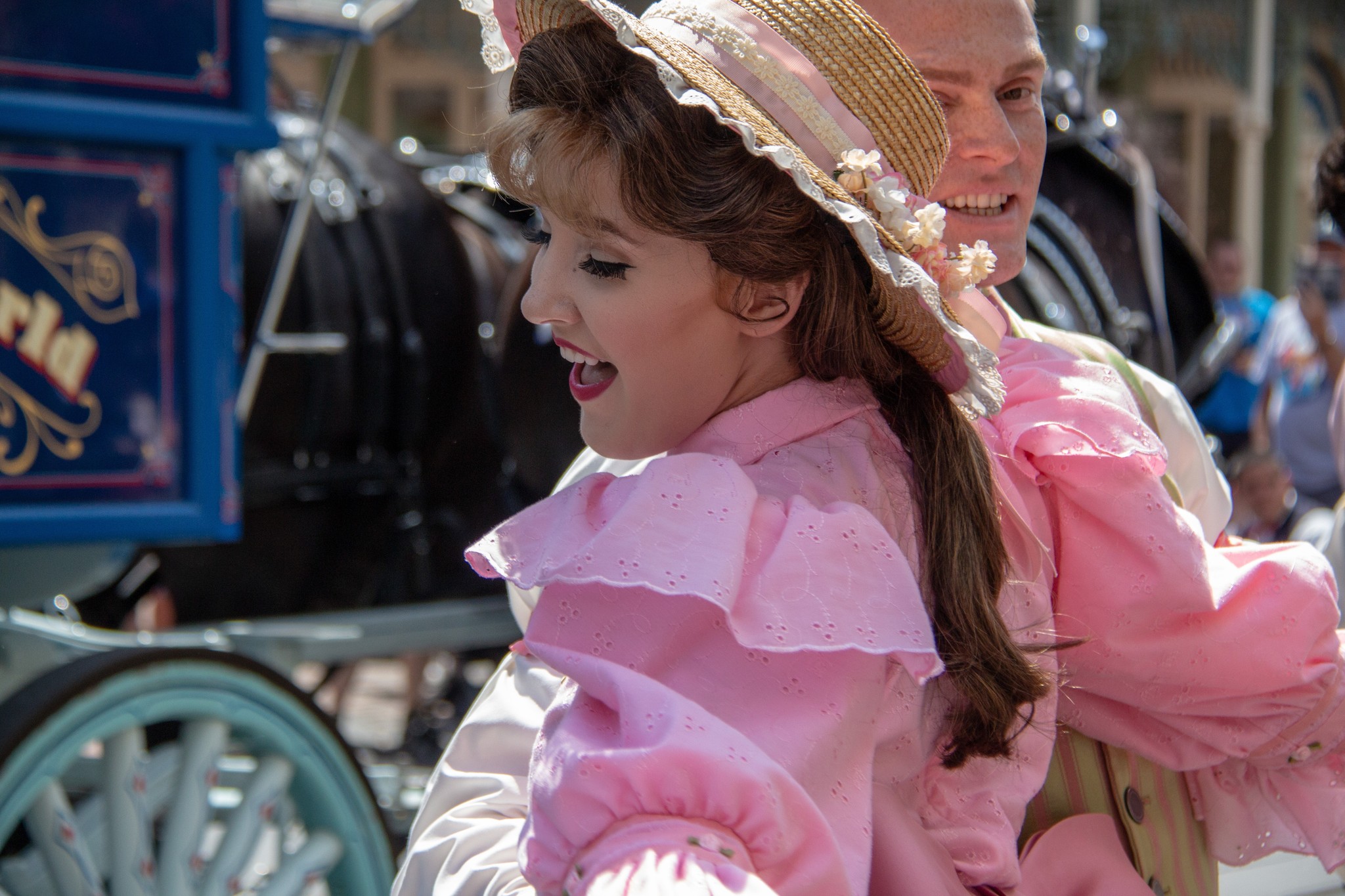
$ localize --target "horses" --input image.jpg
[72,104,1215,765]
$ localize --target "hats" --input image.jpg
[461,0,1011,420]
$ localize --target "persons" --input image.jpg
[390,1,1345,896]
[1187,130,1345,621]
[845,0,1235,896]
[398,652,487,766]
